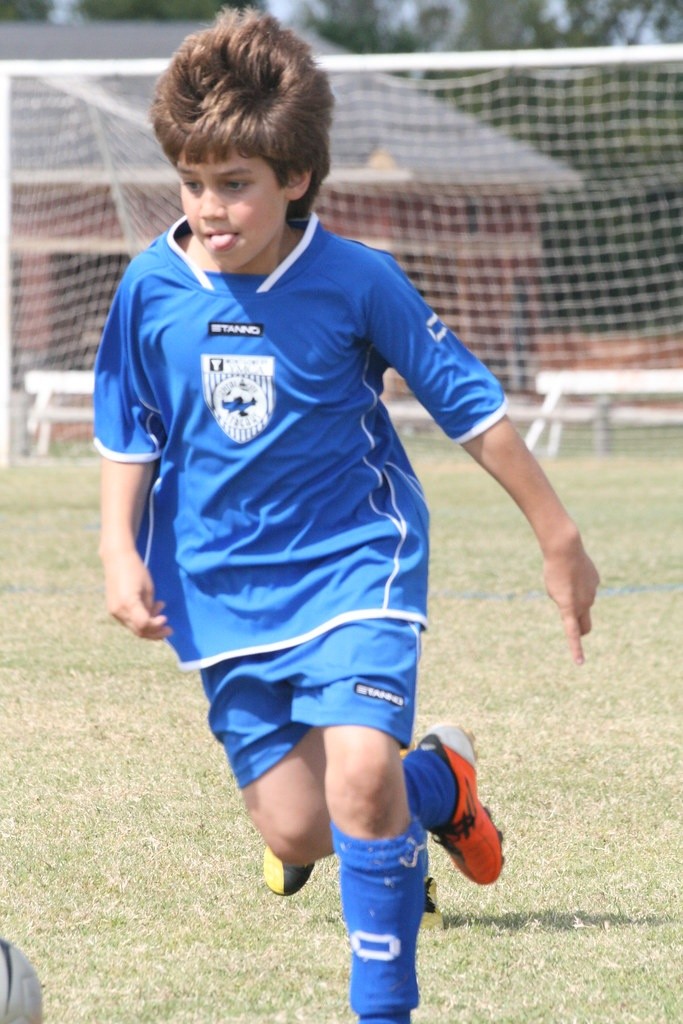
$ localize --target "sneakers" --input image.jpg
[420,723,505,886]
[417,877,444,931]
[265,846,315,896]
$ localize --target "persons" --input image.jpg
[89,2,604,1024]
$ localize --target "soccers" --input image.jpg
[1,939,42,1024]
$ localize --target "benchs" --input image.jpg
[521,367,683,458]
[24,367,98,458]
